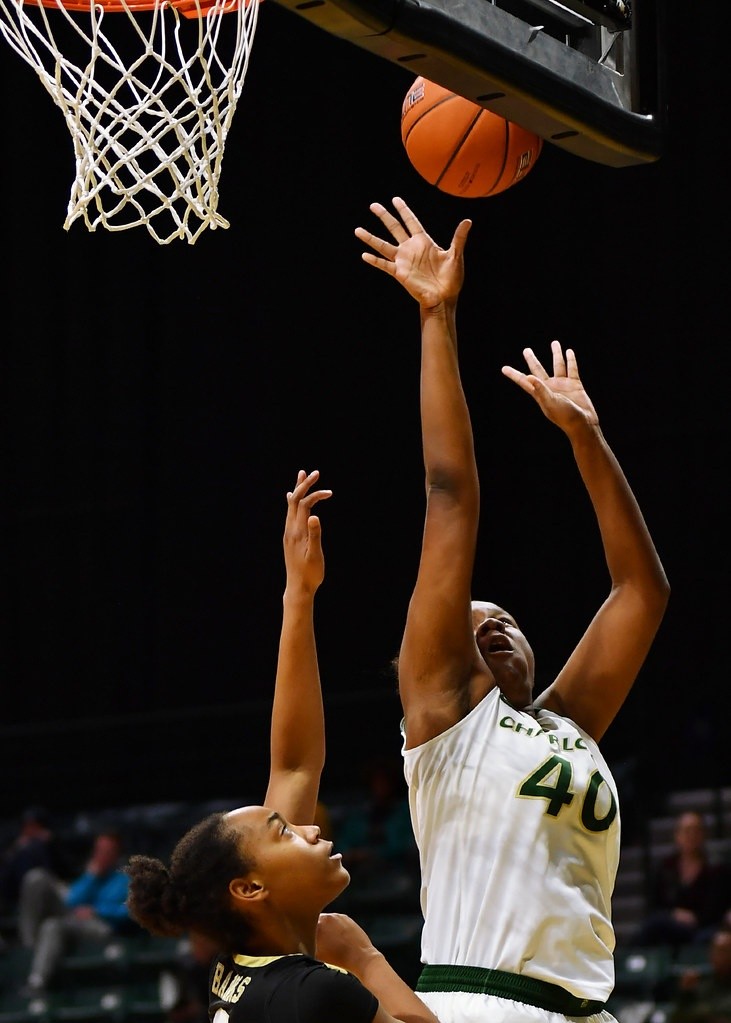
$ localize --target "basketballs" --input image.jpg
[399,73,545,200]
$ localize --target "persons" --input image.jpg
[645,813,731,1006]
[353,197,676,1023]
[122,465,443,1023]
[3,805,141,992]
